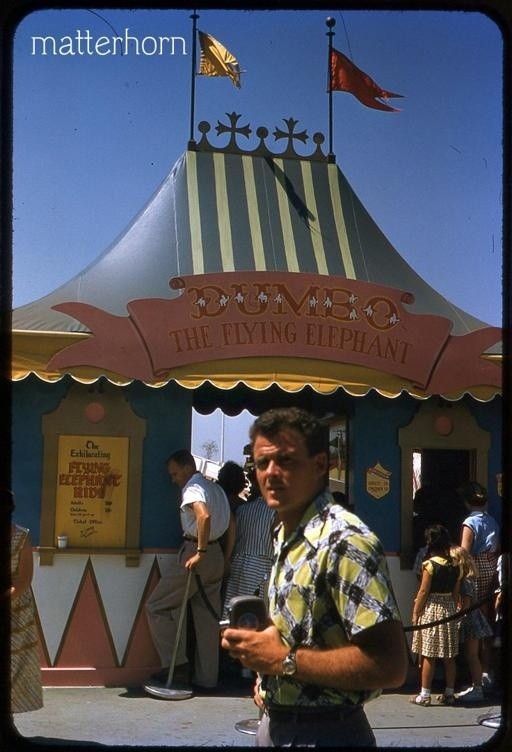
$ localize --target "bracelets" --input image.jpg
[196,547,208,553]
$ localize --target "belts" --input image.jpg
[184,535,219,546]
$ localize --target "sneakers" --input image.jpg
[459,686,485,703]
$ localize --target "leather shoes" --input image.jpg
[150,662,193,682]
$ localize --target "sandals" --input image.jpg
[436,694,457,705]
[409,693,432,707]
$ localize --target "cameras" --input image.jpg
[218,595,267,634]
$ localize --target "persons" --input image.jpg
[8,491,47,718]
[208,405,409,747]
[406,522,466,710]
[477,554,503,730]
[206,443,359,694]
[143,449,237,694]
[453,481,502,703]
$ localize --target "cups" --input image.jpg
[56,536,68,548]
